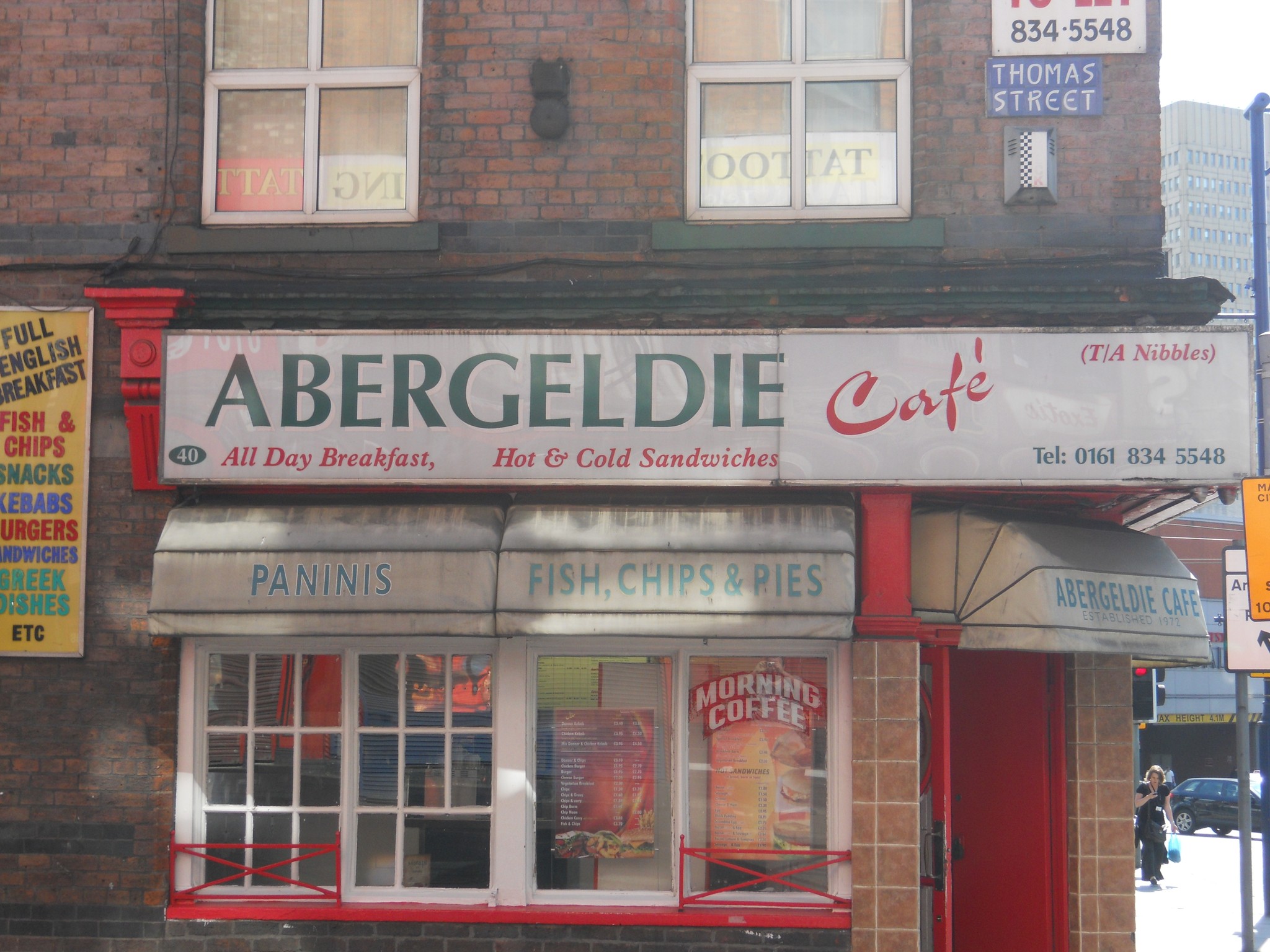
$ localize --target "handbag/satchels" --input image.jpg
[1144,819,1167,844]
[1167,833,1181,864]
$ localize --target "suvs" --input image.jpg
[1171,777,1269,842]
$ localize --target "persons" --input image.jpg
[1139,766,1176,790]
[1135,766,1178,885]
[1230,765,1238,779]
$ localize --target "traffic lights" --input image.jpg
[1131,666,1157,724]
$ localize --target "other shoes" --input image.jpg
[1150,876,1158,884]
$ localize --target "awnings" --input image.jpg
[912,504,1214,672]
[492,499,858,645]
[148,493,504,641]
